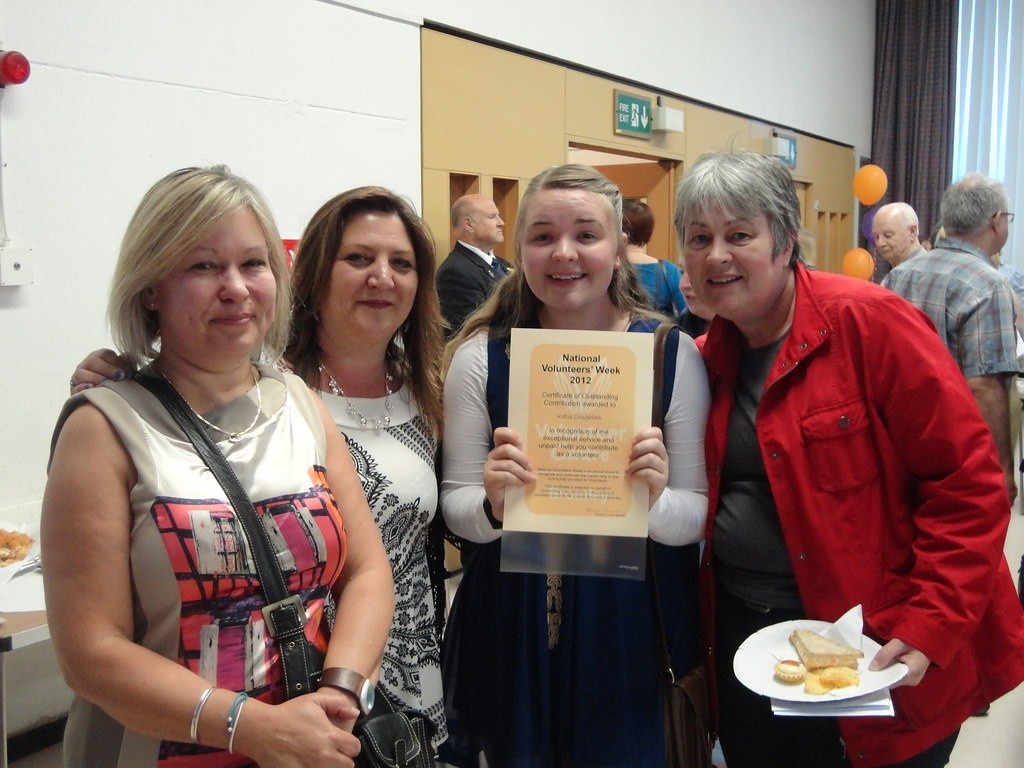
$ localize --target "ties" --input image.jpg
[492,259,505,278]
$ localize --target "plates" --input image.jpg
[733,621,909,703]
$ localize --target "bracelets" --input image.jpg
[188,684,215,743]
[225,689,249,754]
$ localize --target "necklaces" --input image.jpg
[318,356,396,432]
[162,368,263,444]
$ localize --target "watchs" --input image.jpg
[317,666,376,716]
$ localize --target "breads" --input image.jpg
[789,629,865,669]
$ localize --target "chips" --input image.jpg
[805,666,859,694]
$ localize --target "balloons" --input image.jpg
[862,207,881,242]
[839,245,878,282]
[850,162,892,205]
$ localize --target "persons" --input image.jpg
[620,196,684,317]
[38,167,444,768]
[444,162,713,768]
[435,193,515,331]
[672,154,1024,768]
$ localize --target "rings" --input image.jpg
[70,377,75,386]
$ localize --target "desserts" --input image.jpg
[0,528,34,567]
[776,660,807,681]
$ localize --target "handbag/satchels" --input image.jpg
[666,669,713,768]
[280,642,438,768]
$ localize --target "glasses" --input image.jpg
[993,212,1014,222]
